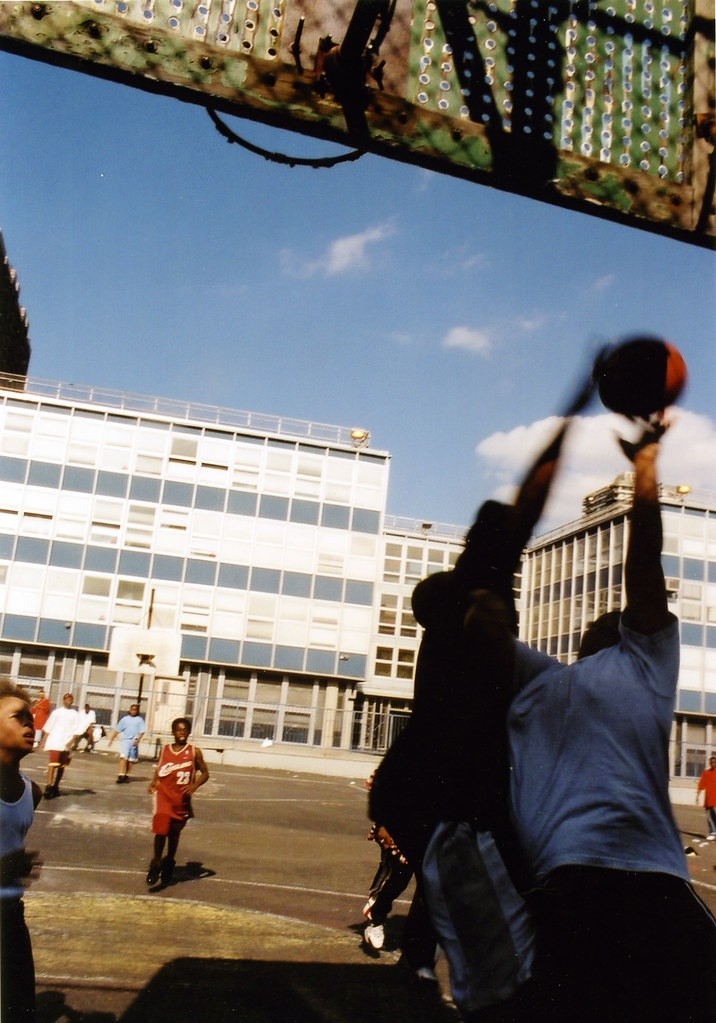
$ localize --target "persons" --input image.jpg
[389,356,716,1023]
[38,692,94,797]
[32,692,51,750]
[147,718,208,883]
[695,757,715,841]
[107,705,146,785]
[0,678,43,1023]
[364,733,414,950]
[73,702,97,755]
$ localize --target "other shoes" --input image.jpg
[84,746,91,754]
[43,785,62,801]
[116,775,130,784]
[33,742,38,747]
[363,914,385,950]
[160,856,176,884]
[706,832,716,840]
[71,745,78,750]
[146,860,163,885]
[398,950,440,990]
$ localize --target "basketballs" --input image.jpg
[597,336,687,418]
